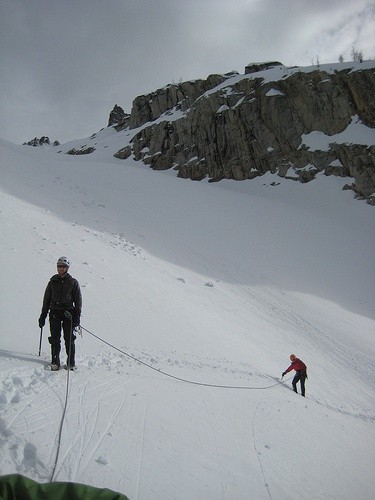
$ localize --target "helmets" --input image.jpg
[57,257,70,267]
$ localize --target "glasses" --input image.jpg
[57,265,66,268]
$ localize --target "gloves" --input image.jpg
[39,316,46,328]
[282,373,285,376]
[73,316,81,327]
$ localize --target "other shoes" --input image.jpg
[50,363,61,371]
[70,365,76,371]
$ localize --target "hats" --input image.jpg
[289,354,295,361]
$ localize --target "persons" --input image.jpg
[39,256,82,371]
[282,354,307,397]
